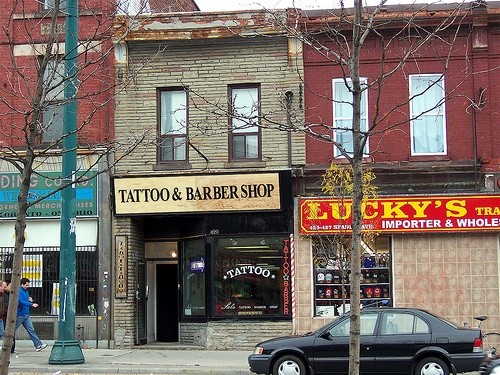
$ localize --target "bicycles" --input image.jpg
[473,316,500,357]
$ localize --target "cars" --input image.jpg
[248,307,486,375]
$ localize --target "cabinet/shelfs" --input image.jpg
[314,253,390,316]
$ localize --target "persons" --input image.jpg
[0,279,7,338]
[15,278,48,352]
[4,281,15,353]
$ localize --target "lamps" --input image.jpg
[229,237,238,246]
[257,237,267,245]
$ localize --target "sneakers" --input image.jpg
[36,343,48,352]
